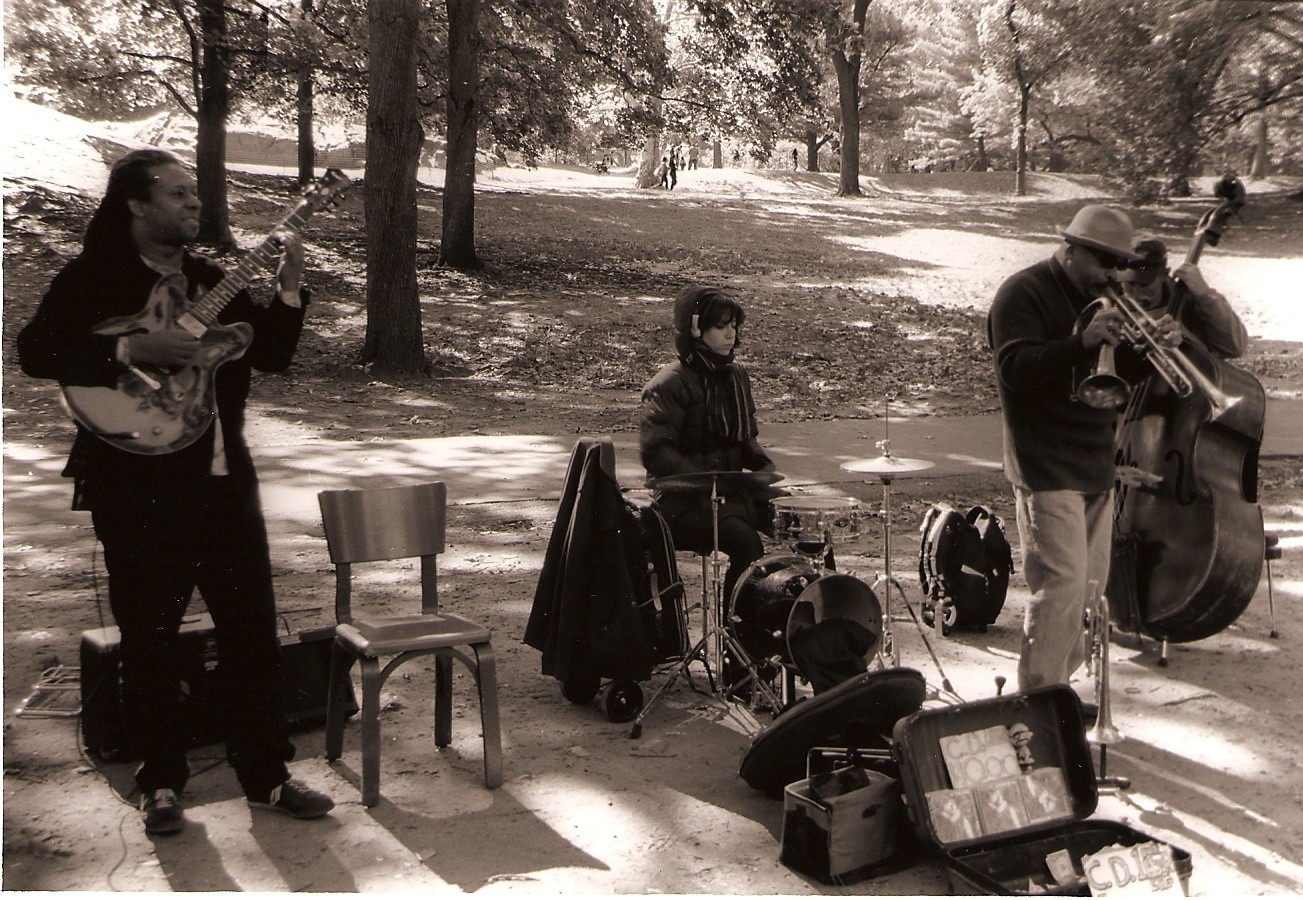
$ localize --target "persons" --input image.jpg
[669,155,677,190]
[601,151,613,174]
[987,206,1181,692]
[17,152,334,834]
[638,284,780,685]
[659,157,669,191]
[670,145,699,170]
[792,149,798,171]
[1118,235,1250,489]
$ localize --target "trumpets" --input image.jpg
[1082,578,1126,747]
[1104,277,1245,427]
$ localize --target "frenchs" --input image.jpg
[1071,295,1133,413]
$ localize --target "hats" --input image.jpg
[1115,236,1169,284]
[1055,203,1146,262]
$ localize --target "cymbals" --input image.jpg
[839,455,937,474]
[642,471,786,496]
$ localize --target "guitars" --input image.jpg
[62,166,358,457]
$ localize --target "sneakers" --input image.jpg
[248,765,335,820]
[141,787,185,834]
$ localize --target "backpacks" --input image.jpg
[917,502,1016,634]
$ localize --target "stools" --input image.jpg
[658,526,721,695]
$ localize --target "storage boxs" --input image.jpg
[779,766,919,887]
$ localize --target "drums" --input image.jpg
[767,496,865,548]
[727,550,885,684]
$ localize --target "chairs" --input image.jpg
[318,481,504,806]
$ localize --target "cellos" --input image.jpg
[1109,172,1268,666]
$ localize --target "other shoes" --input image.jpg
[1080,702,1099,726]
